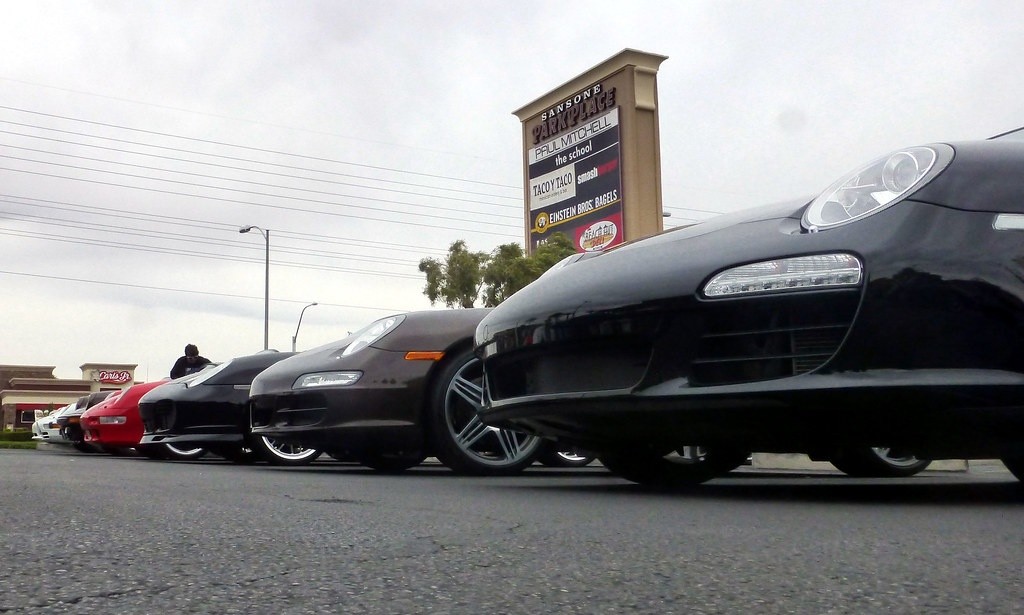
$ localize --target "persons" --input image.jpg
[170,344,211,379]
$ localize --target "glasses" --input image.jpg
[186,355,199,359]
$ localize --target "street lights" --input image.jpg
[292,301,318,352]
[239,224,269,350]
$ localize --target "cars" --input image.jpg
[473,127,1024,490]
[31,349,323,466]
[248,309,598,476]
